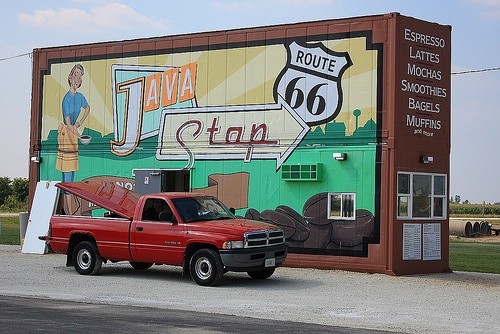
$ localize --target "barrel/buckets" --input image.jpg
[19,212,28,245]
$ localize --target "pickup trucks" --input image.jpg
[38,178,288,286]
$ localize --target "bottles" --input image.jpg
[21,237,24,246]
